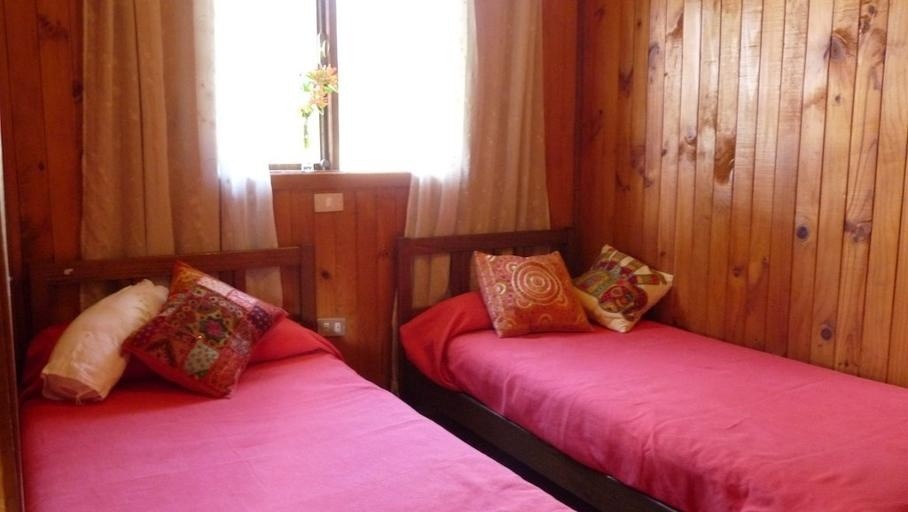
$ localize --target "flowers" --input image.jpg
[296,63,340,146]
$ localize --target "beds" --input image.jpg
[2,244,576,512]
[391,225,906,512]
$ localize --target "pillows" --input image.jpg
[37,277,171,400]
[119,260,287,402]
[570,243,674,333]
[470,249,595,341]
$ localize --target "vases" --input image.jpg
[298,117,316,174]
